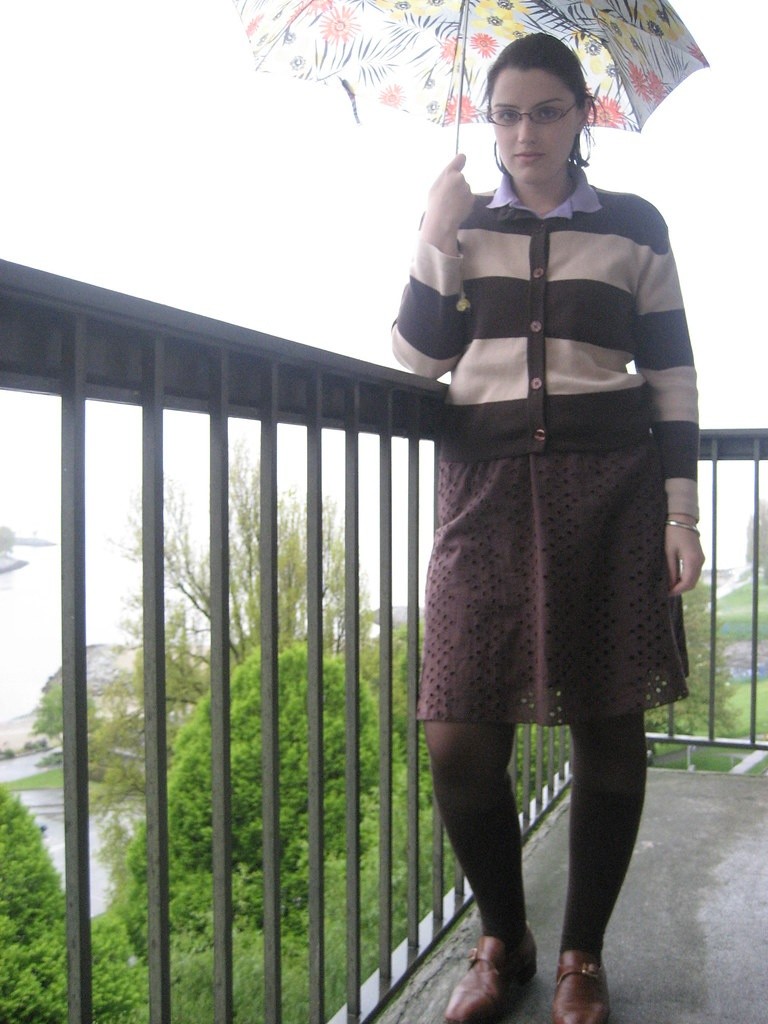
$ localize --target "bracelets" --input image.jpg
[665,520,700,536]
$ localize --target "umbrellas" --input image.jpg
[232,1,711,157]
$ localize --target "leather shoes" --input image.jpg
[552,949,610,1023]
[445,926,537,1024]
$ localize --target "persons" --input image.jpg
[390,32,705,1023]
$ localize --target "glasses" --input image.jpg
[487,93,580,127]
